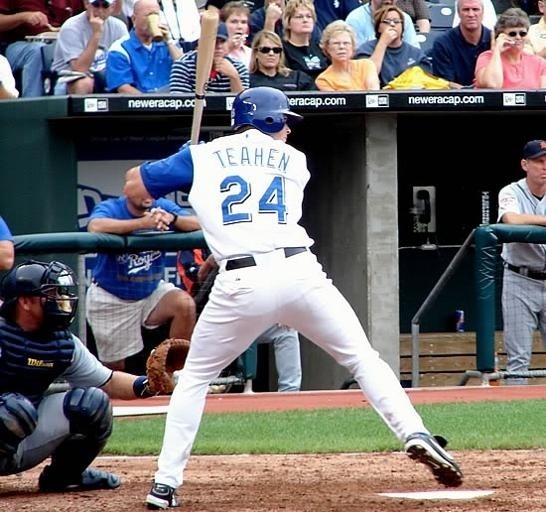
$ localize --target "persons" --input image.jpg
[83,162,203,375]
[176,239,220,312]
[259,322,302,393]
[123,84,466,510]
[494,139,546,387]
[0,258,193,494]
[0,0,545,104]
[0,214,17,305]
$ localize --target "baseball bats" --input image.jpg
[190,10,219,145]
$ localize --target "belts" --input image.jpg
[503,261,546,280]
[225,246,308,271]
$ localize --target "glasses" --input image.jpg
[380,18,403,24]
[91,1,111,8]
[254,46,284,54]
[502,29,528,37]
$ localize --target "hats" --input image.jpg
[216,22,229,41]
[522,140,546,159]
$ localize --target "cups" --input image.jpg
[147,15,164,42]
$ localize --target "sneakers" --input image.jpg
[37,464,121,494]
[146,483,181,511]
[403,432,464,488]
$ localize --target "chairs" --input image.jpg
[39,43,58,96]
[413,4,455,54]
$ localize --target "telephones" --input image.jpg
[414,186,436,233]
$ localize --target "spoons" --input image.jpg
[47,23,61,32]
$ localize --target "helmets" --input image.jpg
[0,260,80,331]
[229,85,305,134]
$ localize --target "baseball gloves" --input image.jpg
[146,336,190,394]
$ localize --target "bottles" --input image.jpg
[456,310,464,332]
[488,351,503,385]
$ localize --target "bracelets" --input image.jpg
[169,210,179,226]
